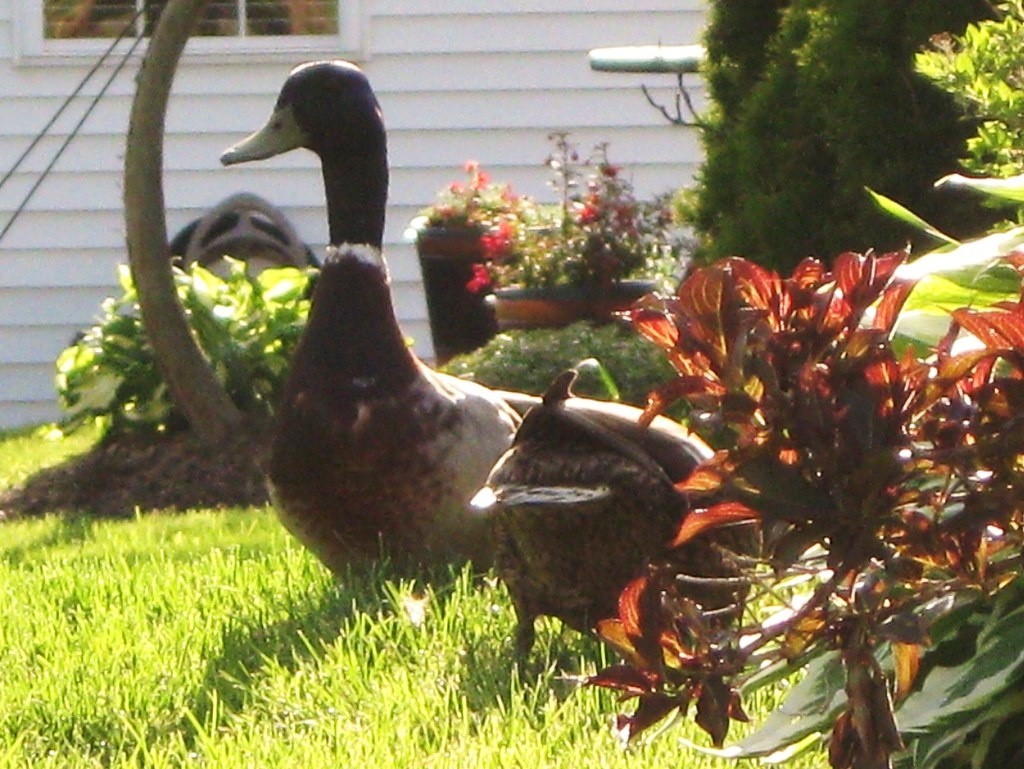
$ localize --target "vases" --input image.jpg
[411,212,588,368]
[491,280,663,335]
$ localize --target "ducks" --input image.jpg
[212,53,763,677]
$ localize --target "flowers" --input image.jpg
[415,122,688,297]
